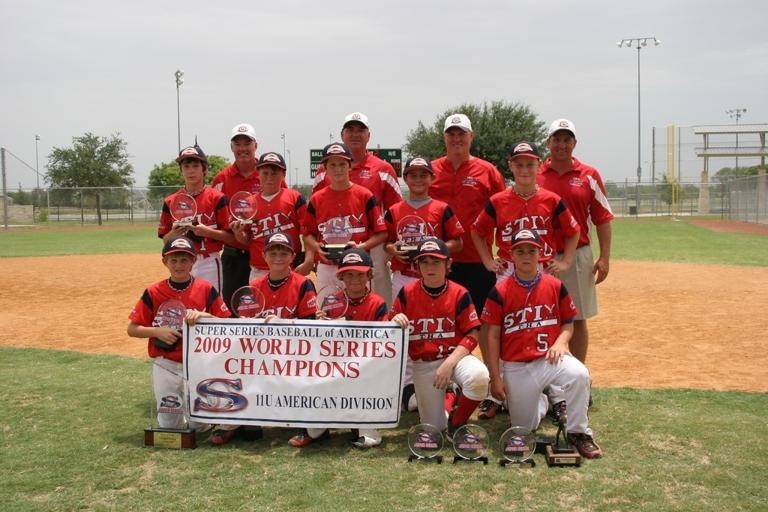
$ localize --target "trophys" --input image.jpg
[143,391,197,449]
[498,426,537,467]
[543,402,580,467]
[452,424,490,465]
[155,299,187,351]
[407,423,444,464]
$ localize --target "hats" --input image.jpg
[547,119,577,141]
[510,141,540,161]
[256,152,286,170]
[343,112,369,128]
[444,114,473,133]
[163,236,195,257]
[264,233,295,254]
[403,157,433,175]
[413,239,449,260]
[336,248,372,274]
[231,124,256,141]
[320,142,352,164]
[510,230,541,250]
[176,147,206,162]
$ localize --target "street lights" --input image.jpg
[174,69,186,186]
[33,132,41,188]
[617,35,663,213]
[723,107,748,182]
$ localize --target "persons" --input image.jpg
[127,235,232,434]
[387,237,490,449]
[157,112,614,425]
[208,232,318,445]
[287,248,389,448]
[479,228,603,460]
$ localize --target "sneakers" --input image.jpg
[478,400,502,419]
[567,433,602,459]
[288,435,312,447]
[207,429,234,447]
[553,401,567,423]
[446,423,466,443]
[444,380,462,395]
